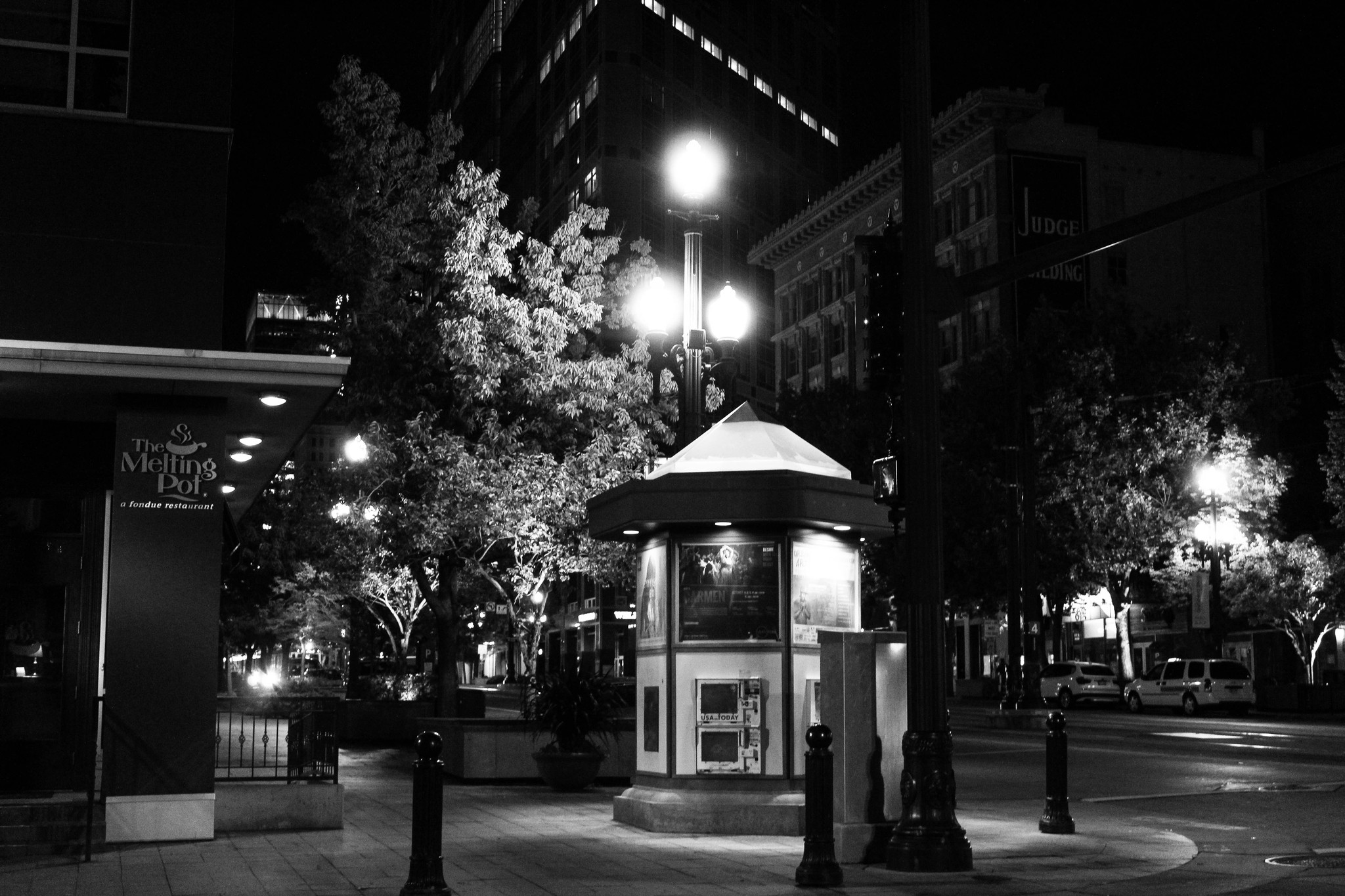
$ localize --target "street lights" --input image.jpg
[468,604,485,684]
[632,132,749,446]
[331,432,380,698]
[1194,455,1234,658]
[529,590,547,673]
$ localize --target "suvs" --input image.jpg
[1040,660,1120,708]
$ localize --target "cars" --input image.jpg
[291,668,343,688]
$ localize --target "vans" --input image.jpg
[289,658,323,668]
[1125,659,1256,714]
